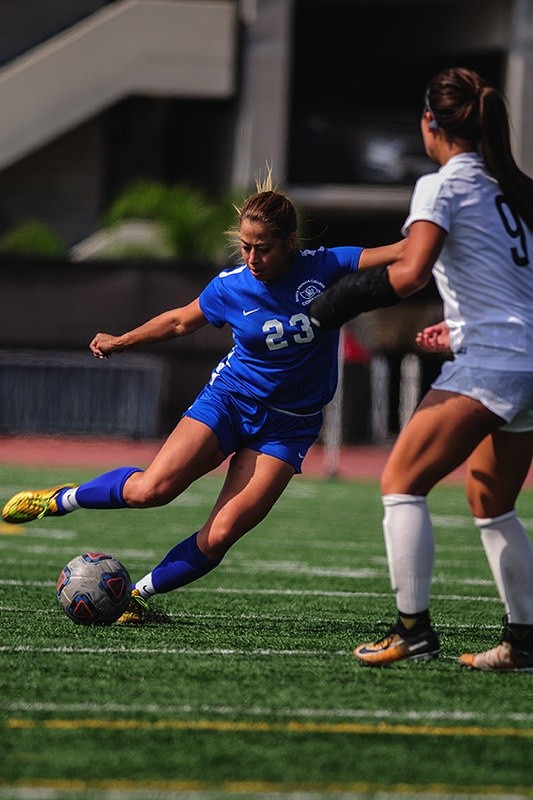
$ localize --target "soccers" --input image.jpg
[57,552,131,627]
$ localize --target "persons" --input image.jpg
[0,191,410,621]
[310,67,533,670]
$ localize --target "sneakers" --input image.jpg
[117,591,150,623]
[1,483,80,523]
[353,617,443,666]
[459,618,533,676]
